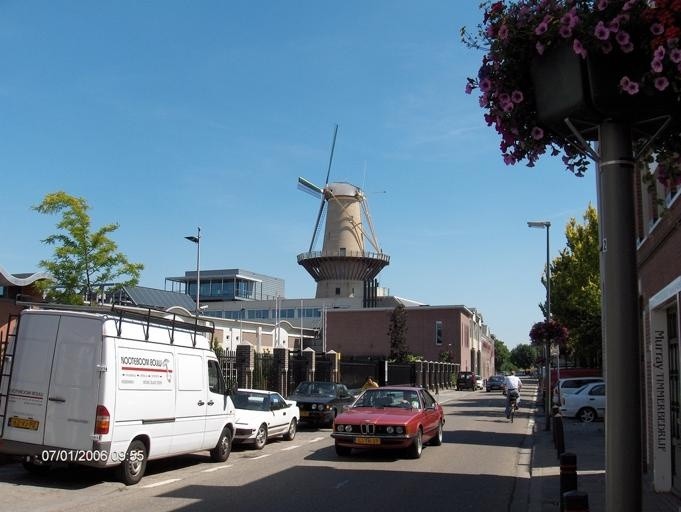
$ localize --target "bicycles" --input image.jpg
[504,391,521,423]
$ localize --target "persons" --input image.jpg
[361,376,379,392]
[503,370,523,418]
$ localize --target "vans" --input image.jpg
[457,371,477,392]
[1,292,241,485]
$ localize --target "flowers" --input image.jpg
[530,321,569,346]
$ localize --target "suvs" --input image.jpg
[552,376,606,407]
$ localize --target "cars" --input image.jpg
[284,380,357,429]
[558,381,606,424]
[485,374,508,392]
[230,387,303,451]
[330,384,447,459]
[474,374,484,389]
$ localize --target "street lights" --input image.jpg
[183,226,203,314]
[527,221,554,432]
